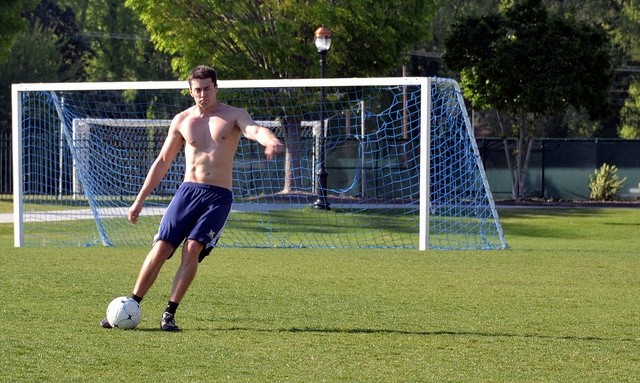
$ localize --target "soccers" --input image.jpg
[105,296,142,329]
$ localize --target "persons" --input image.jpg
[100,64,285,330]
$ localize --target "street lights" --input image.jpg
[312,26,334,209]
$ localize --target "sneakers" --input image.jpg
[100,316,110,327]
[160,310,180,332]
